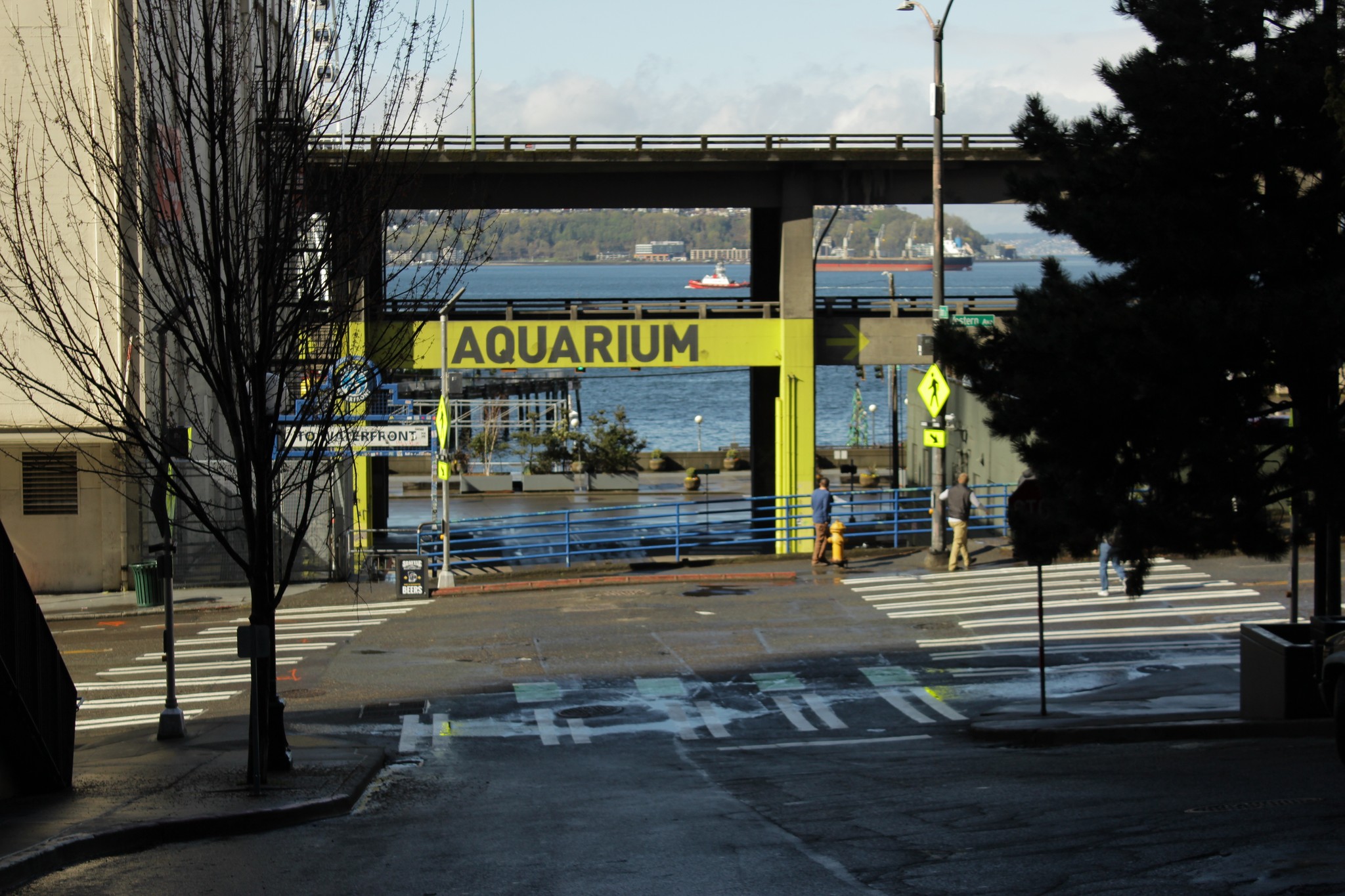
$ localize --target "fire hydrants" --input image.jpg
[826,519,851,566]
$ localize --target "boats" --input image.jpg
[813,217,972,272]
[688,262,752,289]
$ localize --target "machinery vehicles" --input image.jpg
[899,219,918,258]
[870,224,887,258]
[834,223,855,258]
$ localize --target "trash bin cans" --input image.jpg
[129,561,163,606]
[1240,621,1345,719]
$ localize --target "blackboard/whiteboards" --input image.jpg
[396,555,428,598]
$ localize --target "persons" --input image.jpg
[939,472,990,572]
[1091,534,1128,596]
[810,477,830,565]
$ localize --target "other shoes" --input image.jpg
[968,558,976,565]
[1097,589,1109,596]
[811,558,830,566]
[952,566,962,572]
[1123,579,1129,594]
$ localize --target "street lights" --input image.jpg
[146,294,198,743]
[436,287,470,591]
[869,404,878,446]
[571,419,580,462]
[694,415,703,451]
[898,1,954,568]
